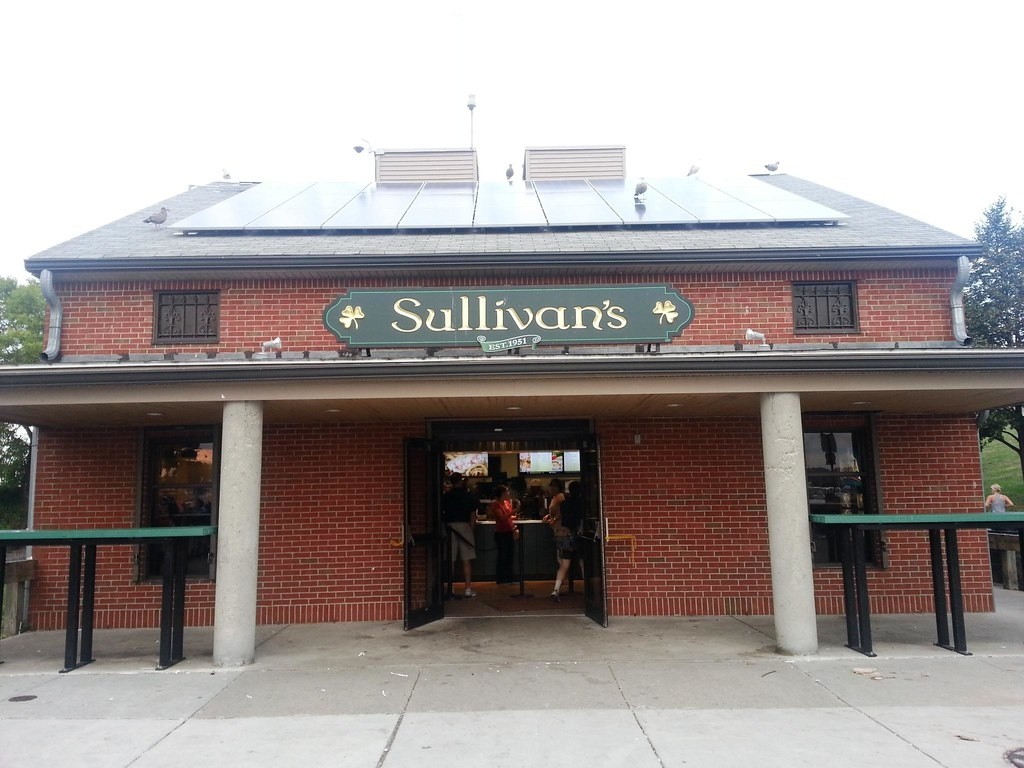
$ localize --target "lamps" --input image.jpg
[259,337,282,355]
[467,94,476,111]
[746,328,765,344]
[352,138,373,154]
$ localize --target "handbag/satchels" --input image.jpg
[512,525,520,541]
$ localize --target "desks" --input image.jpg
[0,527,213,673]
[809,512,1024,659]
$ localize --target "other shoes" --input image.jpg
[550,589,560,603]
[496,577,511,584]
[464,590,476,596]
[511,577,520,582]
[447,586,455,596]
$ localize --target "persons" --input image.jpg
[535,490,550,522]
[442,480,453,494]
[440,472,481,596]
[549,481,586,602]
[526,479,547,496]
[984,483,1015,514]
[542,478,570,585]
[489,485,521,585]
